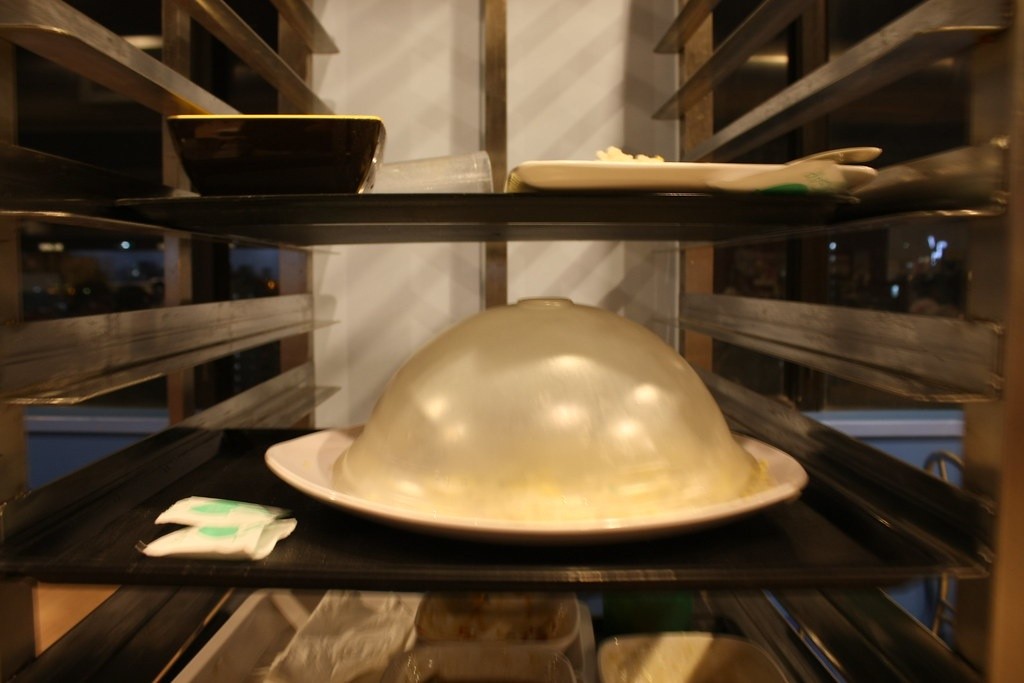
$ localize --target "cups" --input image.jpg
[375,152,493,194]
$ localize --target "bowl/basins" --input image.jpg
[380,644,578,683]
[415,595,580,648]
[168,115,385,194]
[597,633,791,683]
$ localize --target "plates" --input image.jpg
[265,429,807,544]
[506,161,877,193]
